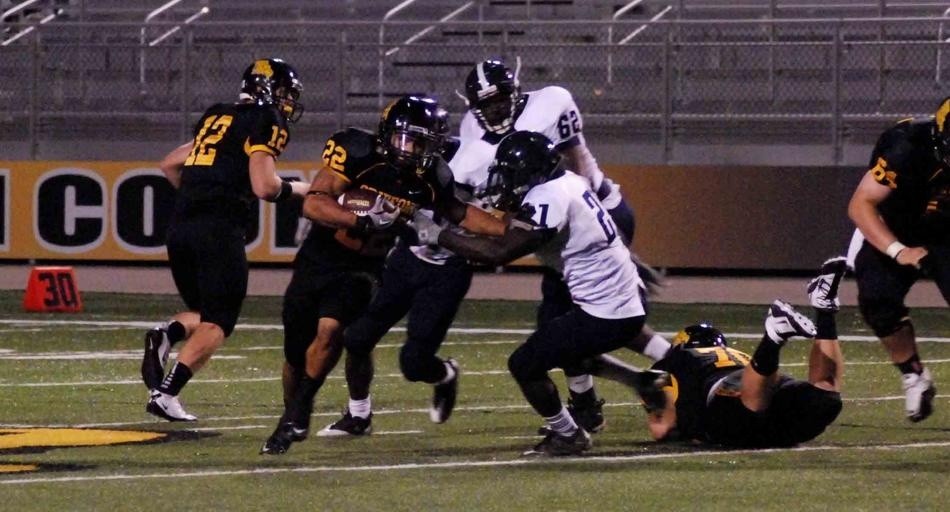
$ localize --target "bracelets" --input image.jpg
[887,241,906,259]
[274,181,292,202]
[355,215,375,239]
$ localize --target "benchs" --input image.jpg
[2,0,950,144]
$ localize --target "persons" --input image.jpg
[644,255,847,450]
[316,110,472,439]
[846,98,949,421]
[448,61,672,434]
[142,59,310,422]
[407,130,678,458]
[258,95,510,455]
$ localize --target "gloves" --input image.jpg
[364,195,402,229]
[400,208,445,247]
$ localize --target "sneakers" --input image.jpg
[259,412,309,456]
[900,367,938,420]
[141,327,171,391]
[537,401,607,434]
[317,410,374,437]
[523,425,593,456]
[762,297,817,347]
[806,257,851,310]
[146,389,199,422]
[639,368,679,440]
[428,357,462,424]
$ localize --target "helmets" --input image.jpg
[380,95,450,175]
[933,98,950,161]
[673,325,729,348]
[238,57,304,122]
[464,60,520,134]
[486,130,563,212]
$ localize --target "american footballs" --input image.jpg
[336,189,396,214]
